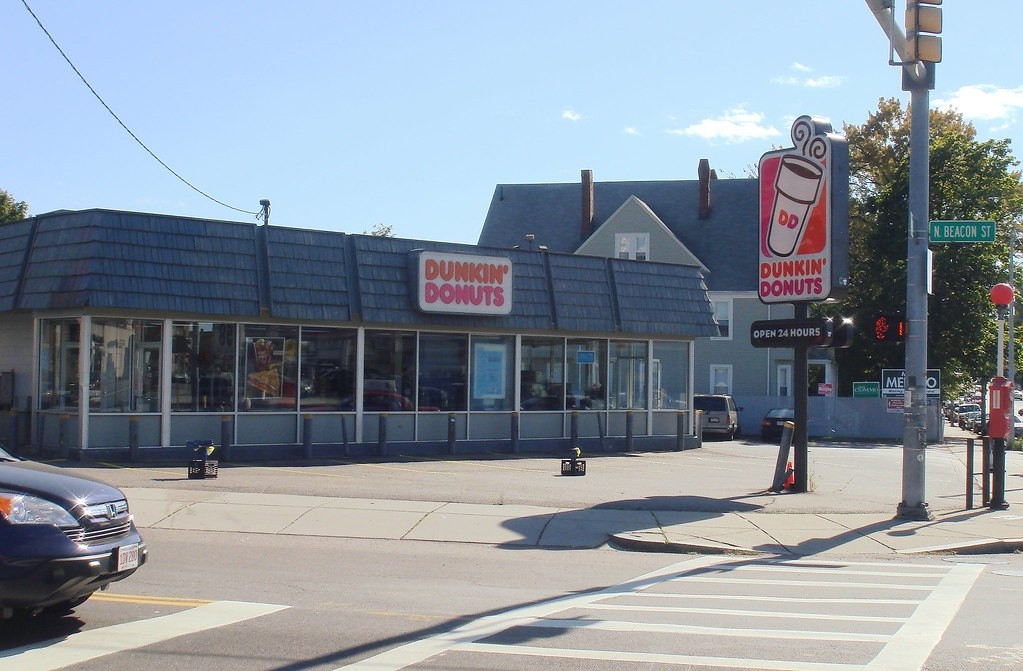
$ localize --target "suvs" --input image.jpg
[692,392,744,442]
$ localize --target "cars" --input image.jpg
[274,367,467,412]
[0,447,148,652]
[199,371,234,407]
[519,389,686,411]
[172,372,191,383]
[760,407,796,444]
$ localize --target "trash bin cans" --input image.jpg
[186,439,214,467]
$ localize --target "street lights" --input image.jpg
[986,284,1015,451]
[942,385,1023,438]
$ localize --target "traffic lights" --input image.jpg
[872,309,906,343]
[903,0,944,64]
